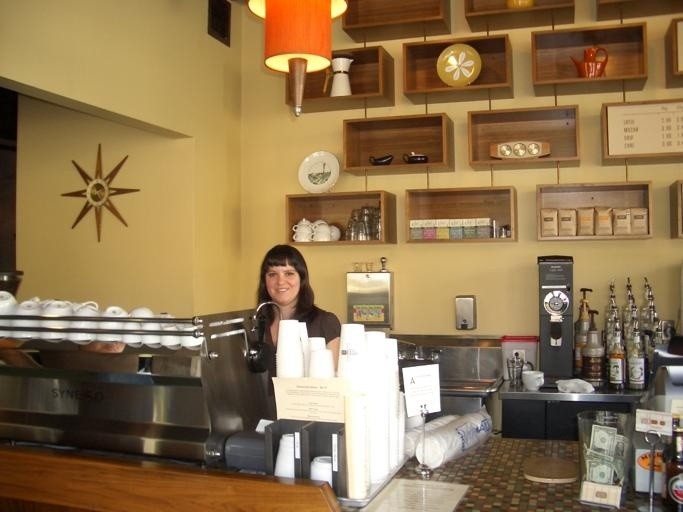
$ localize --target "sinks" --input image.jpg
[439,381,482,393]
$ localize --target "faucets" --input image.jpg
[397,340,417,347]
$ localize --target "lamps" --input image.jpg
[247,0,349,118]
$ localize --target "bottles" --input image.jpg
[664,413,683,511]
[604,274,659,390]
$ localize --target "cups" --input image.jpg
[0,290,204,348]
[415,413,490,469]
[403,393,458,459]
[520,369,545,391]
[573,409,636,509]
[505,353,533,388]
[310,456,333,488]
[291,205,381,241]
[274,431,300,478]
[275,320,385,379]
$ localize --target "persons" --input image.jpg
[252,243,340,377]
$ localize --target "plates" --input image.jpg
[436,44,483,88]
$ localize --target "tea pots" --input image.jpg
[569,46,609,79]
